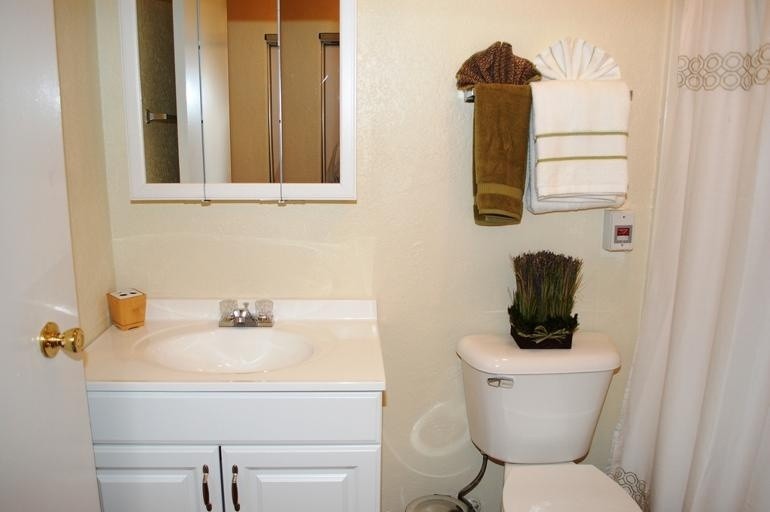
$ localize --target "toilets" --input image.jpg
[456,333,644,512]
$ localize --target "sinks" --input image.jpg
[134,322,312,375]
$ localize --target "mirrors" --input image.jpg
[118,0,358,202]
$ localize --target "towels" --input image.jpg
[533,38,625,82]
[471,83,532,227]
[525,81,630,213]
[458,44,541,91]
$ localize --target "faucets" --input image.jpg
[235,307,257,327]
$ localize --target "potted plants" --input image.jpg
[509,250,583,350]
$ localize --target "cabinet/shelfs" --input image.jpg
[86,382,386,512]
